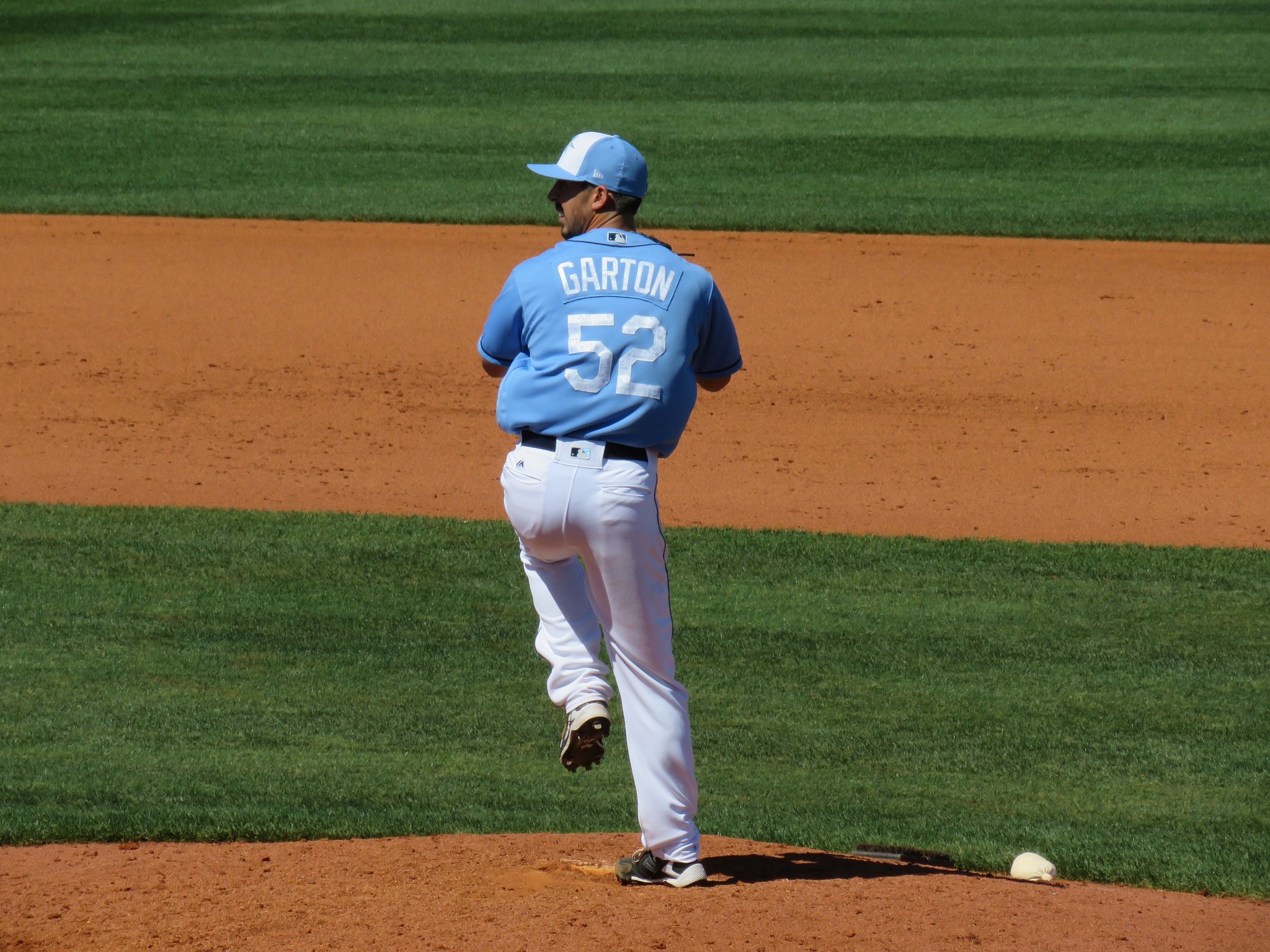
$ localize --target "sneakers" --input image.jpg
[615,846,708,887]
[560,701,611,773]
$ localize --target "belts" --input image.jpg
[521,428,648,463]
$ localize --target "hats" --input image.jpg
[526,131,648,198]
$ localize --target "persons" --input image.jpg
[477,130,743,889]
